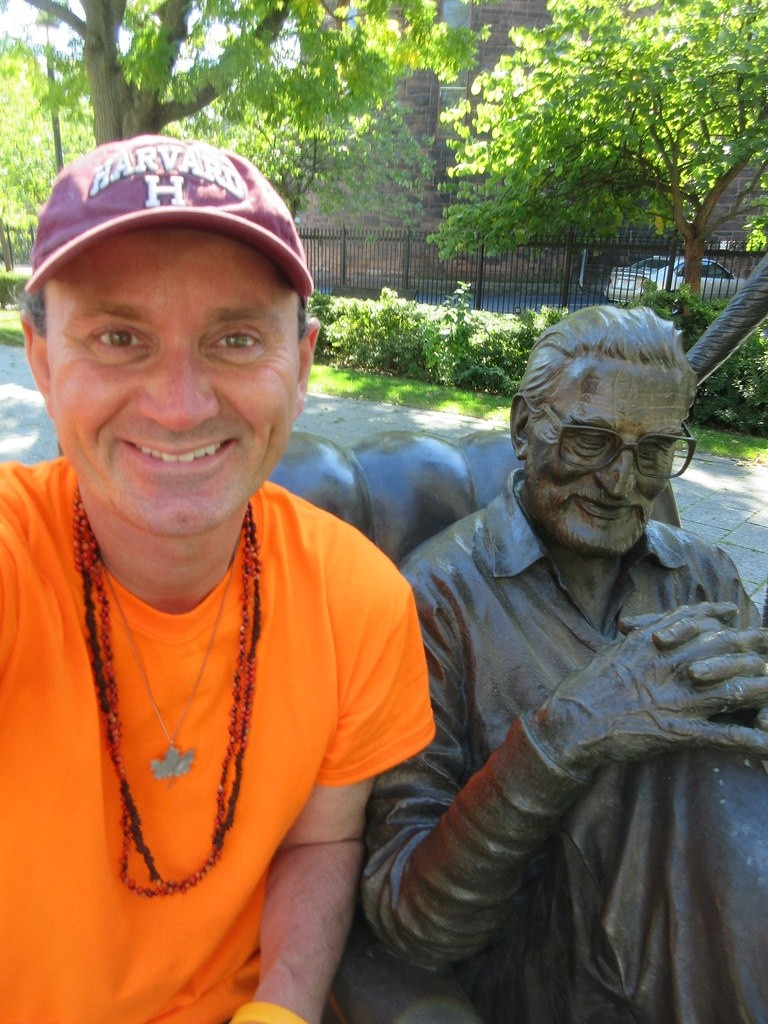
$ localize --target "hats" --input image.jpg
[24,134,317,297]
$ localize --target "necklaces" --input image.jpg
[73,489,264,899]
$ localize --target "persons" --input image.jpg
[357,306,768,1024]
[0,133,436,1024]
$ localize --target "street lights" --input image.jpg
[36,20,65,175]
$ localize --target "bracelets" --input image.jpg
[229,1001,308,1024]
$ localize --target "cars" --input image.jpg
[603,255,746,304]
